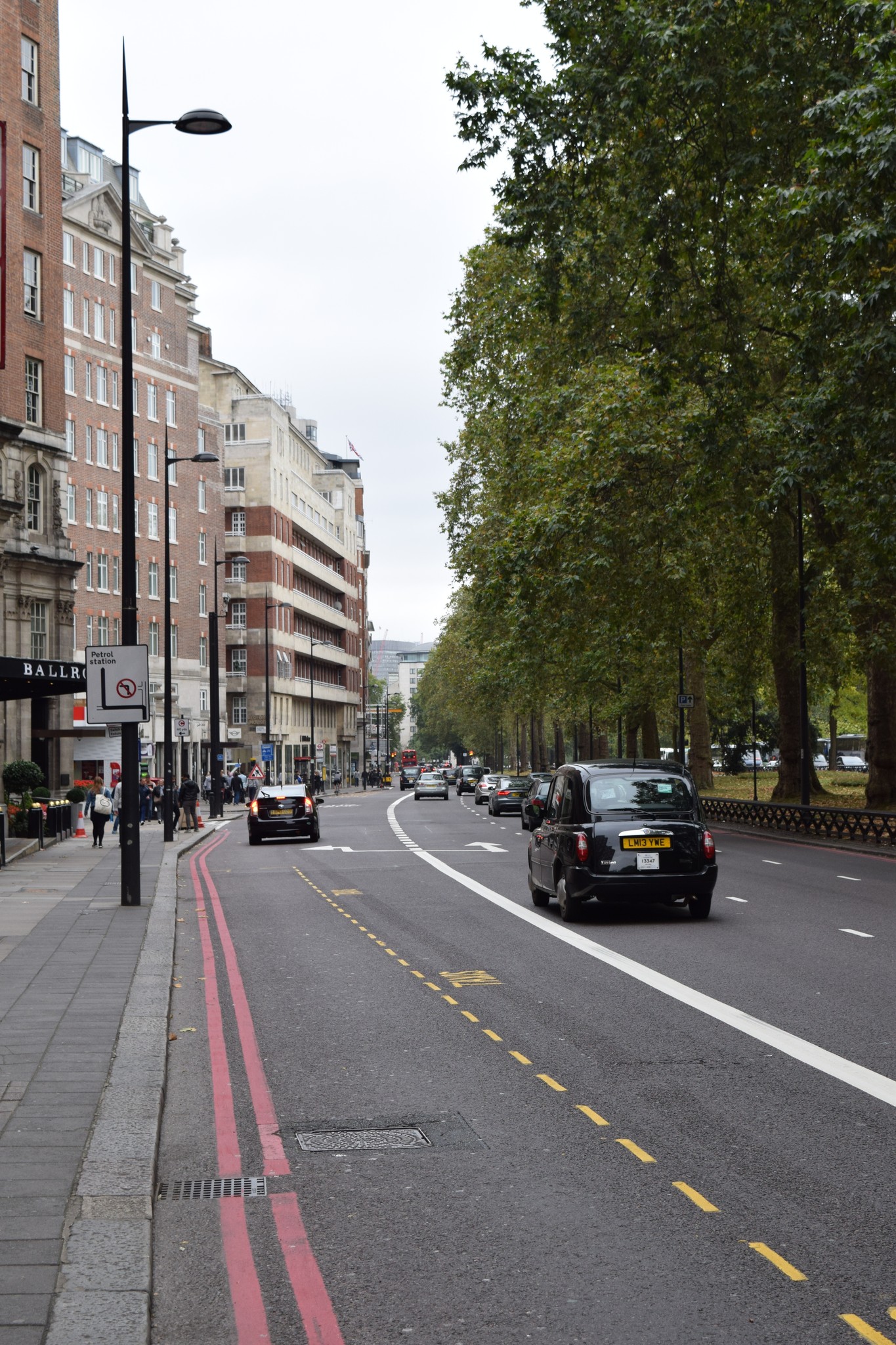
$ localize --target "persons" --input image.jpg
[84,776,114,848]
[111,773,140,847]
[203,770,246,806]
[153,779,164,824]
[353,769,382,787]
[334,771,342,794]
[263,769,303,786]
[178,773,200,833]
[244,777,259,802]
[144,775,156,822]
[139,777,151,825]
[397,766,402,773]
[310,770,327,795]
[173,774,180,832]
[177,777,184,792]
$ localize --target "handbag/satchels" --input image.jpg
[94,787,112,814]
[153,796,162,804]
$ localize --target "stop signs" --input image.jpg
[677,694,694,708]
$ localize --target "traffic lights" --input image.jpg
[471,750,473,756]
[391,753,394,758]
[469,751,471,755]
[394,751,396,756]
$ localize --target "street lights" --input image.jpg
[362,678,380,790]
[161,417,223,844]
[264,585,293,786]
[208,535,252,817]
[115,34,234,907]
[384,686,400,775]
[309,631,333,795]
[384,699,397,739]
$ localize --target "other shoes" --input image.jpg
[224,803,228,805]
[173,828,180,832]
[92,842,97,846]
[158,820,162,824]
[99,844,103,848]
[173,830,176,833]
[141,820,144,825]
[184,829,191,833]
[194,829,199,832]
[112,830,118,834]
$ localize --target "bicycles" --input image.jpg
[334,781,341,796]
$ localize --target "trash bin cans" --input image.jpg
[305,783,313,796]
[381,774,391,786]
[178,808,195,830]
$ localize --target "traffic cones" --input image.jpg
[73,811,89,838]
[196,800,206,828]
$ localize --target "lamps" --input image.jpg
[30,546,40,551]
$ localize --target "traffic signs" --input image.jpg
[173,718,191,739]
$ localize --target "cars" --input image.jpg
[399,749,491,796]
[246,784,324,846]
[473,775,513,805]
[519,776,554,832]
[488,777,534,816]
[414,773,450,800]
[527,772,553,780]
[661,733,869,771]
[524,757,719,925]
